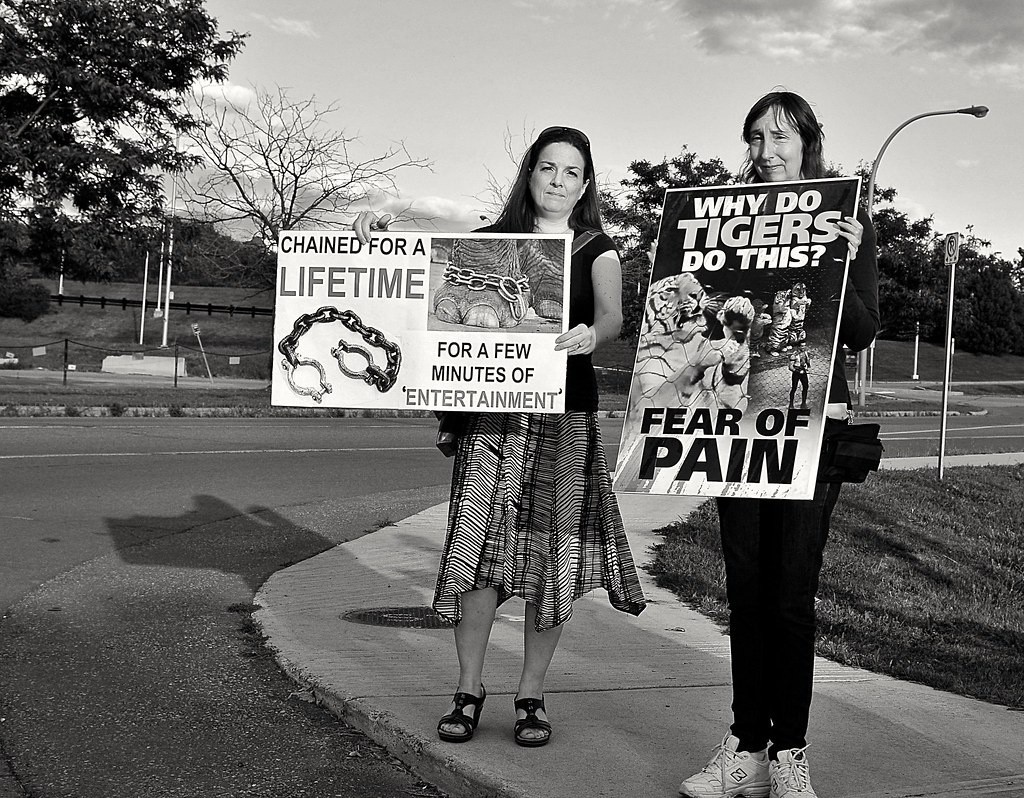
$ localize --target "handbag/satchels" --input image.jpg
[819,416,884,483]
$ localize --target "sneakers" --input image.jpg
[679,731,771,798]
[769,742,818,798]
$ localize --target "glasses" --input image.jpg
[538,127,590,150]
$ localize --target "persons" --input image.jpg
[644,90,881,798]
[352,126,647,748]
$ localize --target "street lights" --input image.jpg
[159,119,212,347]
[911,233,943,380]
[858,104,989,404]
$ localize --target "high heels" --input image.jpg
[436,682,486,742]
[513,692,553,747]
